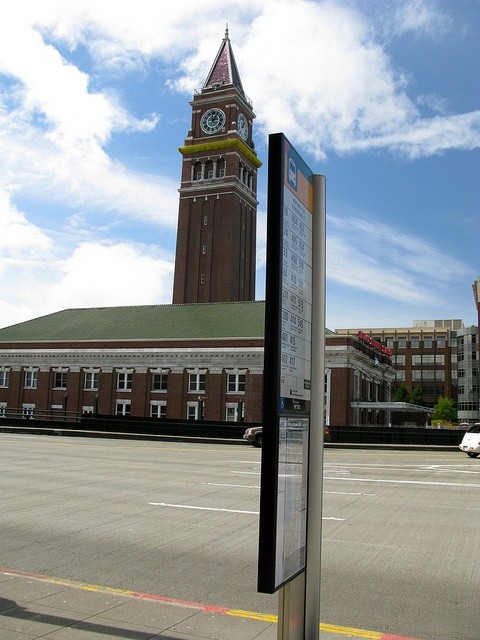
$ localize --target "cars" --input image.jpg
[244,423,331,448]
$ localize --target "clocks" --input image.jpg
[199,107,226,135]
[237,112,249,142]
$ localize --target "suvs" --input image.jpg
[458,422,480,457]
